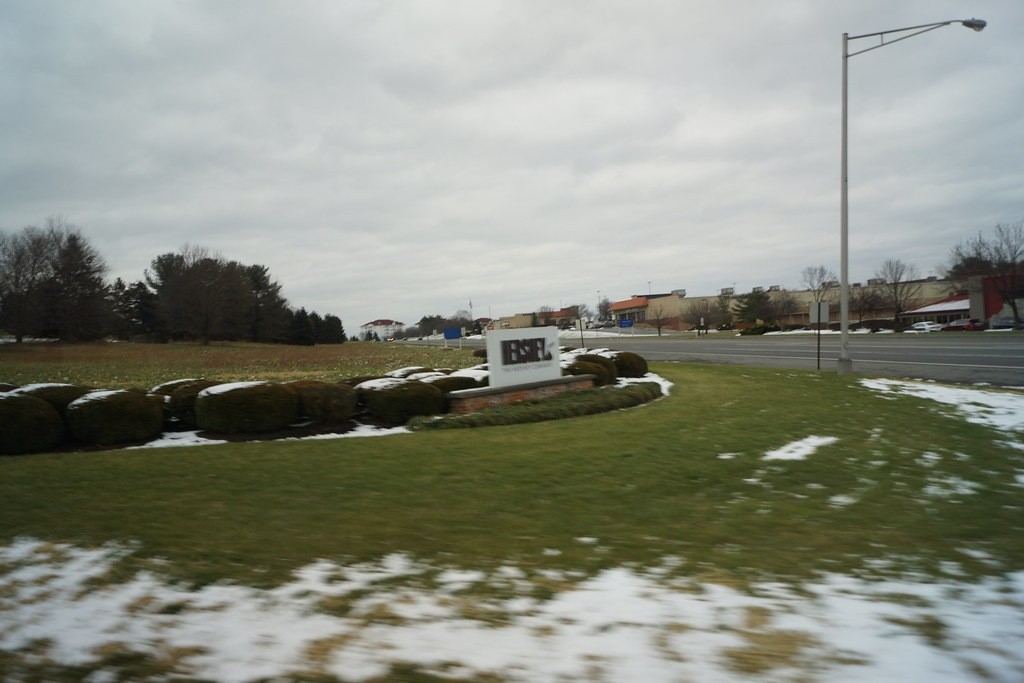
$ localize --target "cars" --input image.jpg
[991,315,1024,330]
[911,321,943,332]
[687,325,710,331]
[941,318,984,332]
[718,324,733,330]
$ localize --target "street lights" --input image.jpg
[839,16,988,376]
